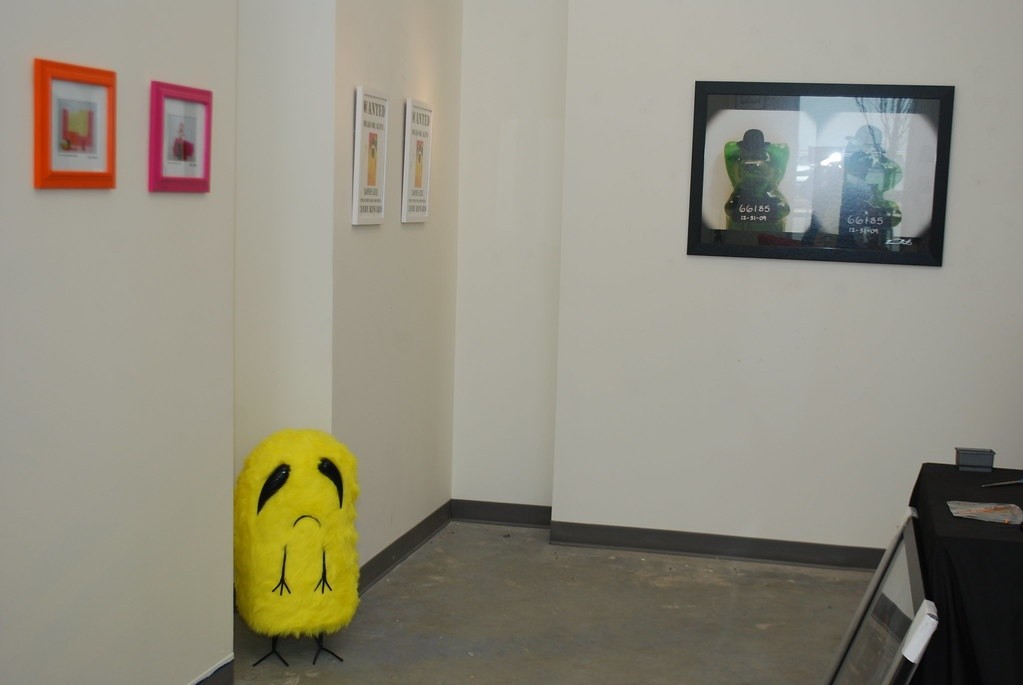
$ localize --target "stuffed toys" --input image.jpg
[234,428,361,667]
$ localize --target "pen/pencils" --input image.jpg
[982,479,1023,487]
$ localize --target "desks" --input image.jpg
[911,461,1023,684]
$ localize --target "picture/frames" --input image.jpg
[145,81,214,191]
[32,57,116,189]
[400,97,434,223]
[352,86,390,225]
[687,80,954,267]
